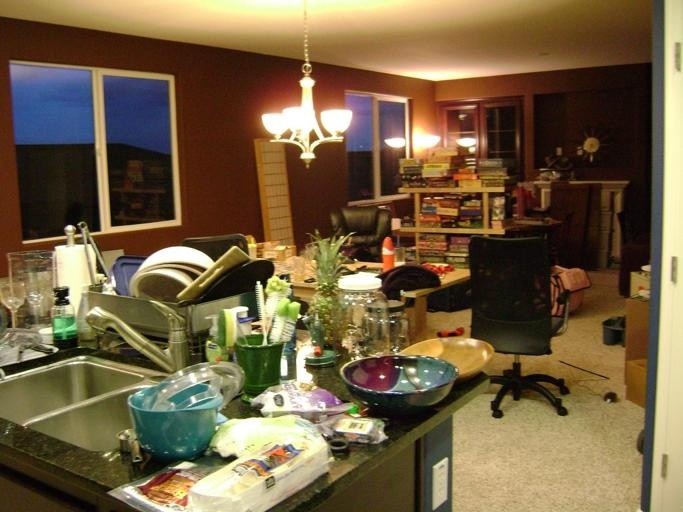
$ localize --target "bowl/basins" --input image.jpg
[132,246,213,303]
[341,354,457,411]
[127,381,221,457]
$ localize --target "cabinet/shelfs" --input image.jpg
[395,90,522,270]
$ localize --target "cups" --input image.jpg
[234,342,284,396]
[6,249,58,328]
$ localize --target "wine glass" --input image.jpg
[22,274,47,329]
[0,276,23,331]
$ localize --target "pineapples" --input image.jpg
[304,226,358,350]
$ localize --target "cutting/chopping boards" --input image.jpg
[177,245,249,302]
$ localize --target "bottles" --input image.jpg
[50,286,78,346]
[335,277,389,357]
[381,236,395,271]
[361,298,411,355]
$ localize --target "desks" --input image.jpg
[535,179,630,271]
[288,259,471,345]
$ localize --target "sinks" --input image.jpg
[23,373,170,450]
[0,355,143,426]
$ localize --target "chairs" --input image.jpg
[467,234,573,419]
[328,206,389,261]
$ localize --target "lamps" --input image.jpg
[259,8,353,167]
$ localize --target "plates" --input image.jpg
[197,257,274,301]
[400,336,493,380]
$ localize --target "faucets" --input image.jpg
[85,301,190,371]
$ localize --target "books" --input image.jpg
[397,145,517,269]
[112,159,172,223]
